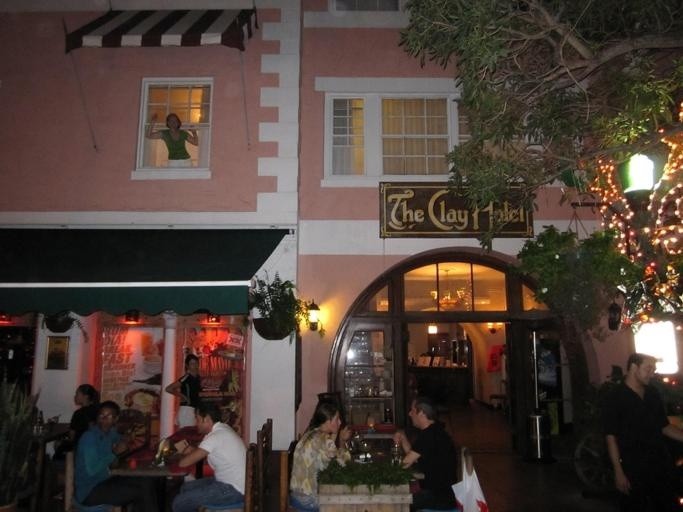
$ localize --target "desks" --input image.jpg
[25,420,68,512]
[107,450,208,512]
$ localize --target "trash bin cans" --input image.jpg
[527,409,553,465]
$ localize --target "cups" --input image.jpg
[373,352,384,366]
[48,418,56,424]
[159,439,169,456]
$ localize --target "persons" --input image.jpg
[604,354,683,512]
[393,396,458,510]
[54,354,247,512]
[146,113,198,169]
[289,403,353,512]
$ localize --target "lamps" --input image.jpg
[303,299,323,331]
[122,308,140,322]
[605,299,623,333]
[616,151,654,194]
[429,267,465,312]
[204,313,223,326]
[425,323,437,338]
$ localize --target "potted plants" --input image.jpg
[240,269,301,346]
[35,310,88,345]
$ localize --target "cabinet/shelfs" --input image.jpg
[342,328,396,435]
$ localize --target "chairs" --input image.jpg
[277,439,299,512]
[455,444,476,511]
[198,440,260,512]
[254,416,274,512]
[60,450,121,512]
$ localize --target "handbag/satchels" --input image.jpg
[451,448,488,512]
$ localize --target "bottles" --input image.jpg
[35,410,44,435]
[366,411,375,429]
[384,408,392,423]
[390,439,401,464]
[345,369,386,397]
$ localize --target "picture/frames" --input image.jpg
[43,334,71,370]
